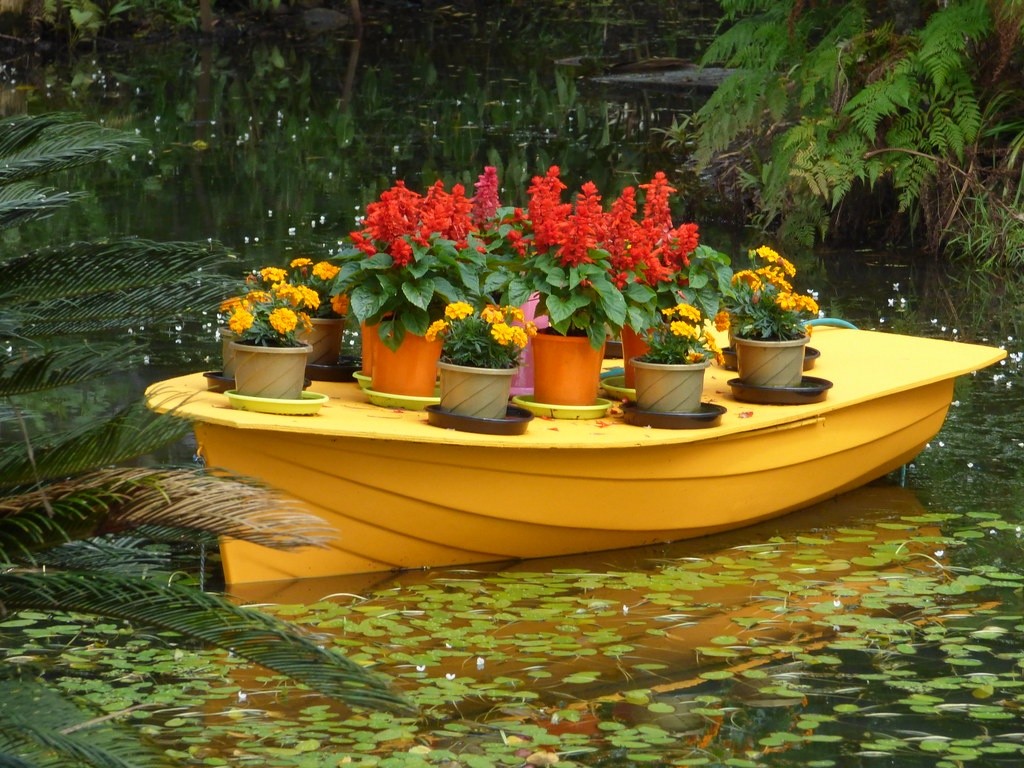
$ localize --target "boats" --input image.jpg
[141,314,1007,602]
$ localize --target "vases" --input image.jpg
[206,308,834,434]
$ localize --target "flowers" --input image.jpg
[217,165,821,360]
